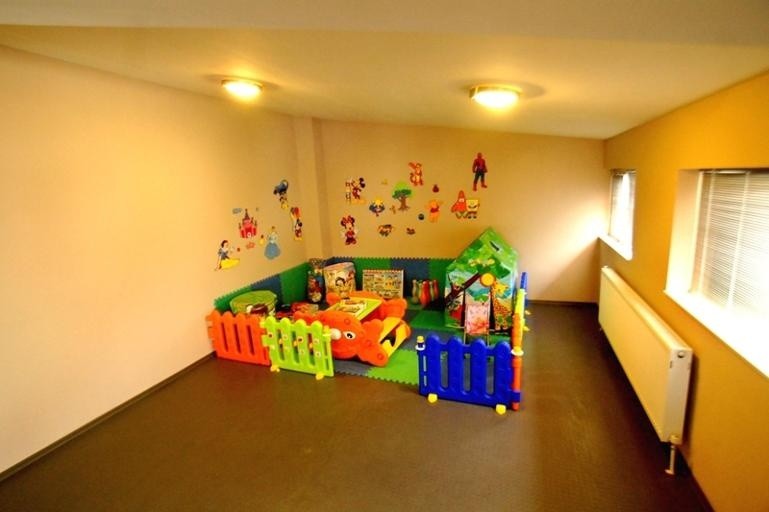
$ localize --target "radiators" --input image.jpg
[596,264,695,476]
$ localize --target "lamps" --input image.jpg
[218,75,265,103]
[467,82,523,115]
